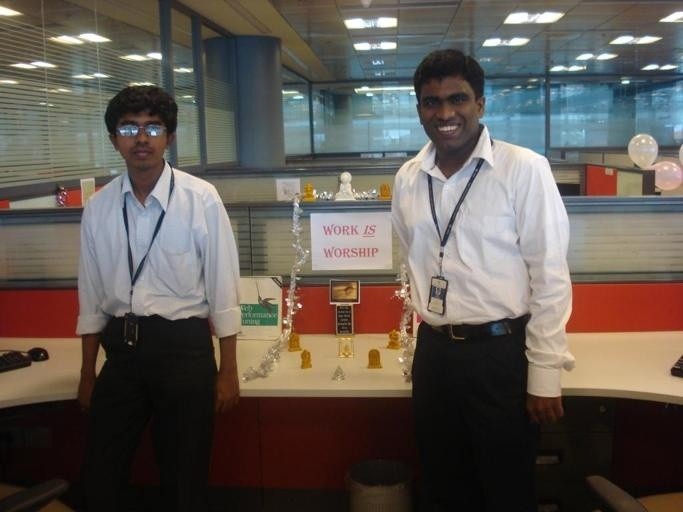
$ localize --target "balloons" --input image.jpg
[627,133,659,168]
[649,161,683,192]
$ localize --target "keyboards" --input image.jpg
[0,351,31,372]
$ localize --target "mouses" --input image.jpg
[28,347,49,361]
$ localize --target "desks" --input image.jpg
[0,331,682,418]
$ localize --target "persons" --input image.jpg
[75,86,243,511]
[390,49,576,512]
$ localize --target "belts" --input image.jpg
[422,317,528,341]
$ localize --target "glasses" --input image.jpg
[116,124,168,138]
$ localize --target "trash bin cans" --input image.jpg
[346,459,408,512]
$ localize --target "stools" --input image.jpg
[580,471,681,512]
[0,470,77,512]
